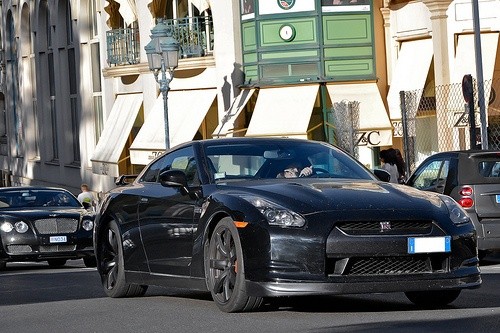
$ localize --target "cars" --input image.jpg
[1,185,98,270]
[404,148,499,252]
[91,136,484,314]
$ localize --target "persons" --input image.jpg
[77,183,96,211]
[277,164,313,178]
[379,148,407,183]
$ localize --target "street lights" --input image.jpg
[142,19,182,151]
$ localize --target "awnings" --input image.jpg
[325,82,395,147]
[90,93,144,178]
[386,38,433,137]
[128,86,217,166]
[211,83,320,140]
[451,32,500,128]
